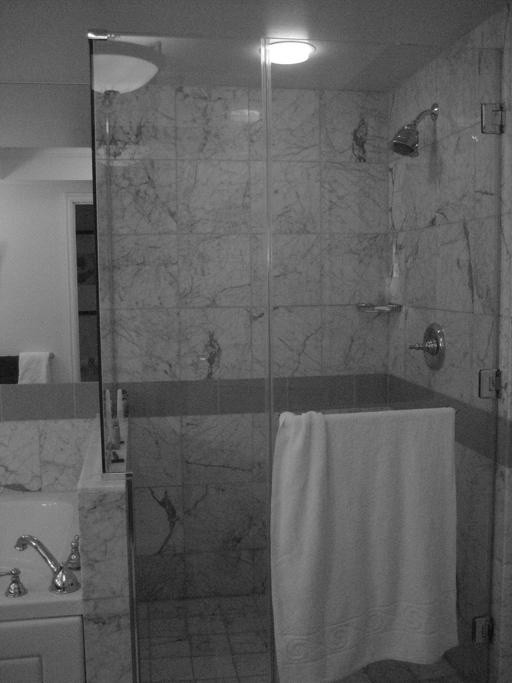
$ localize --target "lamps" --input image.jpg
[91,37,168,109]
[258,39,316,65]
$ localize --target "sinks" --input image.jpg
[0,490,84,621]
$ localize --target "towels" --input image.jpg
[17,350,53,385]
[267,405,462,680]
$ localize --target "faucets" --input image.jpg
[14,534,81,595]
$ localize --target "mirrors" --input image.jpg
[1,143,103,385]
